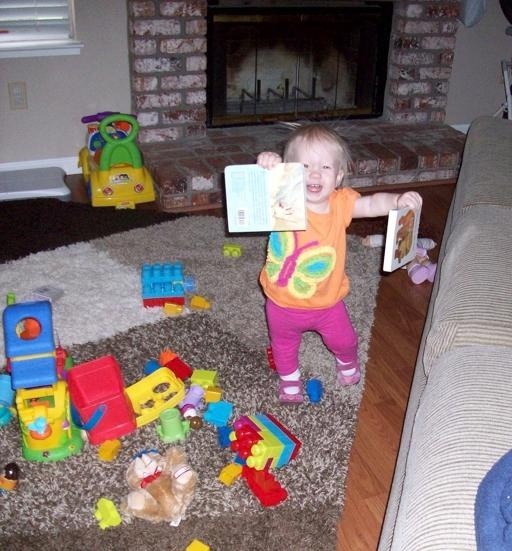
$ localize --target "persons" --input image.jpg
[250,123,425,405]
[364,228,438,282]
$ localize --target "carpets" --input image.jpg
[1,216,385,551]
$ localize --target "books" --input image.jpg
[223,161,310,235]
[382,206,421,273]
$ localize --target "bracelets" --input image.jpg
[397,194,403,204]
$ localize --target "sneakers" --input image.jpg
[278,377,303,403]
[336,359,360,385]
